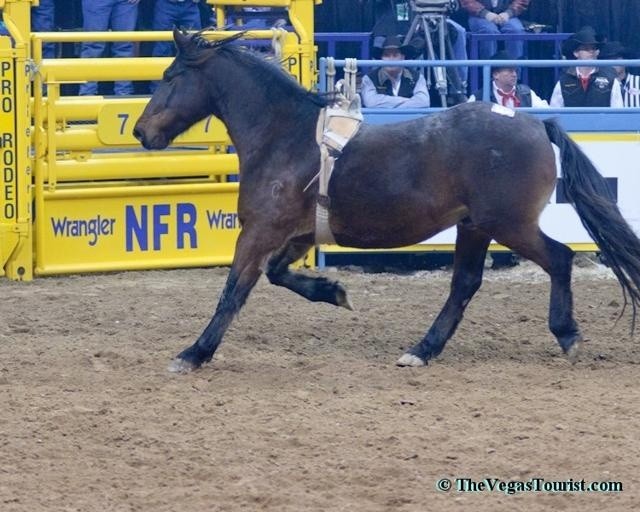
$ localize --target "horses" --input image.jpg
[132,24,640,375]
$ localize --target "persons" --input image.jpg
[0,2,640,112]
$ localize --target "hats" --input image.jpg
[562,31,601,57]
[369,36,417,60]
[490,50,528,78]
[599,41,624,57]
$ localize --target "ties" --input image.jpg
[581,76,588,92]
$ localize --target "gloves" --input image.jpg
[485,12,503,27]
[499,12,509,24]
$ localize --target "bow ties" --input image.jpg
[496,89,521,107]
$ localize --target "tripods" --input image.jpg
[402,13,466,108]
